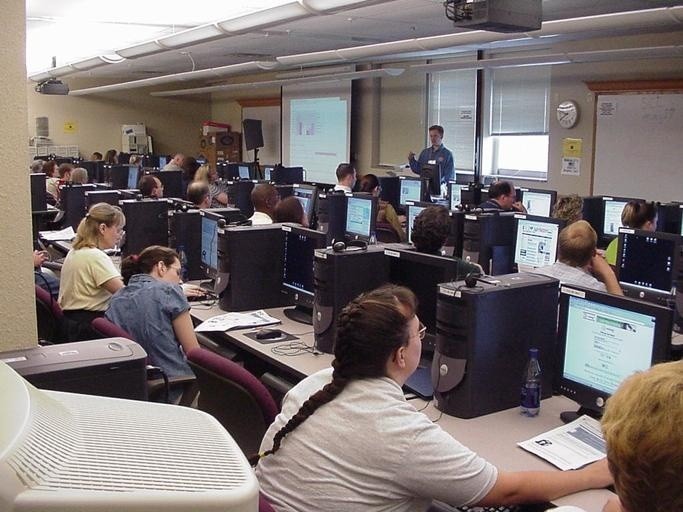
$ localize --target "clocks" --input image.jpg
[556,100,580,130]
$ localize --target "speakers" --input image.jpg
[242,118,265,151]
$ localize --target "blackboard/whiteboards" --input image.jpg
[241,105,282,167]
[589,89,683,206]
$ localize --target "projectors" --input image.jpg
[445,0,541,34]
[35,81,70,96]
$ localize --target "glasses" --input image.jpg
[408,320,426,342]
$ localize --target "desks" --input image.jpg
[183,298,617,511]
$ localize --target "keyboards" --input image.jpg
[433,499,548,511]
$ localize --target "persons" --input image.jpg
[103,149,119,164]
[128,155,141,167]
[527,219,624,297]
[90,151,104,162]
[137,174,164,198]
[58,201,128,336]
[160,152,186,174]
[244,183,283,224]
[353,173,405,239]
[407,126,457,202]
[117,151,131,164]
[32,246,61,300]
[190,165,229,207]
[550,192,584,226]
[111,244,202,379]
[332,164,358,197]
[31,158,90,211]
[248,282,614,512]
[184,181,213,210]
[478,179,528,214]
[273,197,312,228]
[603,198,659,274]
[539,357,682,512]
[406,203,453,259]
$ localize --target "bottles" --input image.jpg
[670,280,677,295]
[519,349,541,417]
